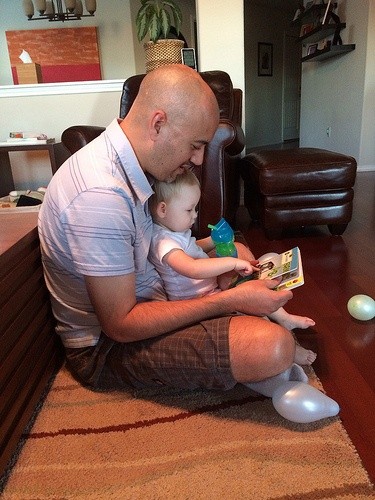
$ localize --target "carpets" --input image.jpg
[0,333,375,500]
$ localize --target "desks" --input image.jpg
[0,213,67,455]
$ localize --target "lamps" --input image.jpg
[23,0,96,22]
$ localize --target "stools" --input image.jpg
[240,147,357,240]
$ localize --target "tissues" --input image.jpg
[14,49,44,85]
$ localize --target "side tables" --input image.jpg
[0,142,71,199]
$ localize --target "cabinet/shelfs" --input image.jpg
[292,0,355,62]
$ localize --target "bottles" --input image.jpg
[207,218,238,258]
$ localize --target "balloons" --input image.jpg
[272,381,341,423]
[347,294,375,321]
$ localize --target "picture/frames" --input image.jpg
[181,47,198,73]
[257,41,274,77]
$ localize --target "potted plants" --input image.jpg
[135,0,185,74]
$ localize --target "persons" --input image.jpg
[38,62,296,395]
[144,168,316,331]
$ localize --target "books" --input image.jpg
[226,247,304,292]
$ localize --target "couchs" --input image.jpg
[61,70,245,233]
